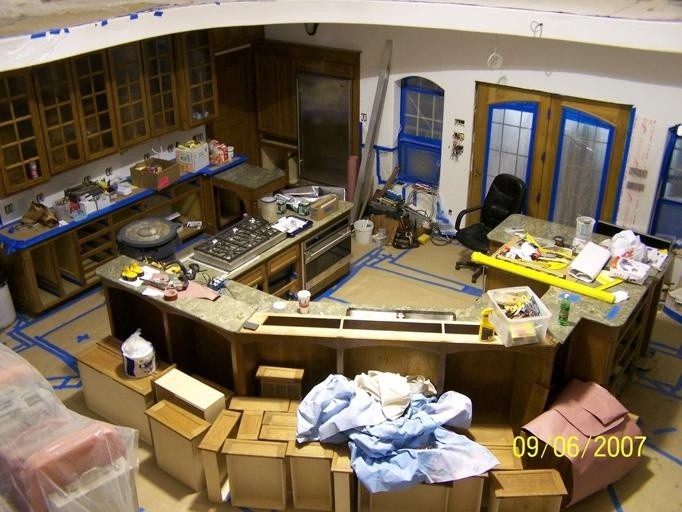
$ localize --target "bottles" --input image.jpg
[28,160,39,180]
[558,294,571,327]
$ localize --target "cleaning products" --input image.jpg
[479,308,495,342]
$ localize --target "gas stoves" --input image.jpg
[193,213,287,268]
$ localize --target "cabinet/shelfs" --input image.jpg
[252,39,362,189]
[0,28,221,200]
[0,145,248,316]
[486,211,666,399]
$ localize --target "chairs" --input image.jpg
[455,168,527,284]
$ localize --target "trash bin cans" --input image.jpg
[116,217,181,265]
[0,269,17,330]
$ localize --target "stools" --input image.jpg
[211,161,287,230]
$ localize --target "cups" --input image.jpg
[296,290,311,314]
[576,216,596,241]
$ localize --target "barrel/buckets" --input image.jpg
[575,216,595,239]
[354,220,374,243]
[52,199,70,219]
[260,196,278,222]
